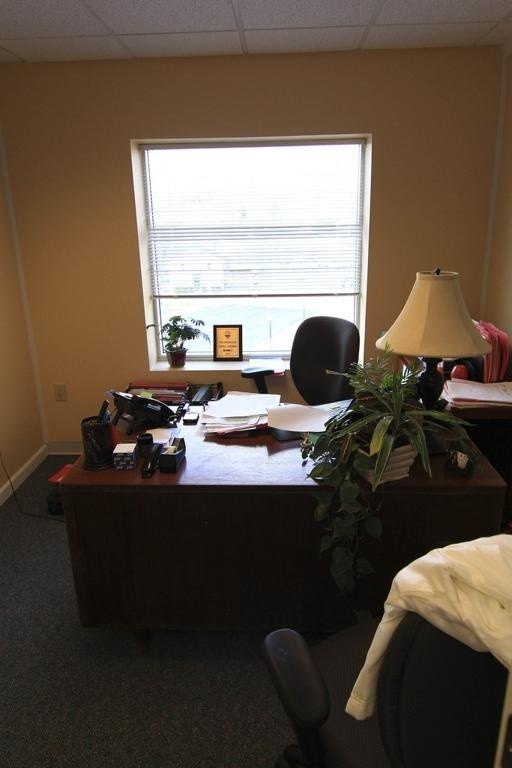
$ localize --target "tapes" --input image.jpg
[168,446,177,454]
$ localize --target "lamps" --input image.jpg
[374,268,492,451]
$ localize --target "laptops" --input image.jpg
[324,384,392,420]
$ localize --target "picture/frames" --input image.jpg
[212,324,244,362]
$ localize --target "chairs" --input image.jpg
[262,529,512,768]
[241,316,361,405]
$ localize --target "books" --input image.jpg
[242,357,286,376]
[441,377,512,412]
[128,380,190,404]
[200,389,282,438]
[355,437,419,484]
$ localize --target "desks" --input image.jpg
[394,352,512,450]
[57,398,508,639]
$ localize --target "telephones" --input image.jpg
[112,391,174,435]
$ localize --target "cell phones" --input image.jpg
[183,411,199,424]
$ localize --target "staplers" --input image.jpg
[141,443,163,479]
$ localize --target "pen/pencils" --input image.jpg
[97,399,111,424]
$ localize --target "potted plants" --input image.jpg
[144,314,213,368]
[302,340,483,595]
